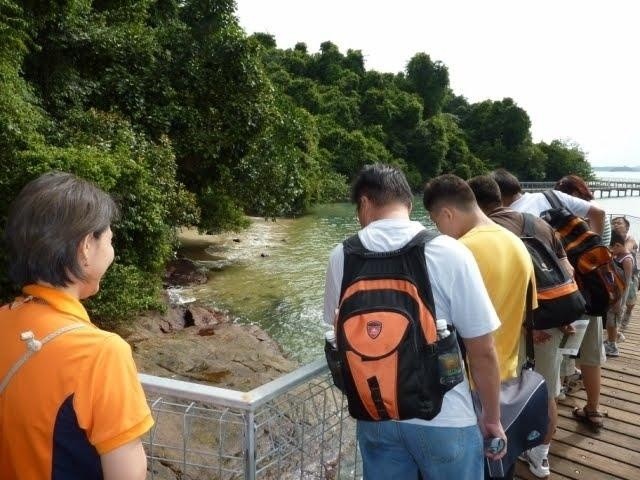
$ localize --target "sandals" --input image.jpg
[571,404,603,428]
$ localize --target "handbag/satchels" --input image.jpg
[472,366,548,479]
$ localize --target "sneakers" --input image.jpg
[518,448,551,479]
[616,332,626,343]
[604,338,619,357]
[560,373,587,394]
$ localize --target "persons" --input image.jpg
[602,229,633,357]
[611,215,639,326]
[465,175,576,478]
[0,171,158,480]
[553,175,614,403]
[488,165,607,436]
[322,161,510,479]
[423,170,539,479]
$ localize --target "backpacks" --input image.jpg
[324,229,466,423]
[519,190,629,330]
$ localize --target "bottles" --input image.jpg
[325,329,345,389]
[433,319,465,386]
